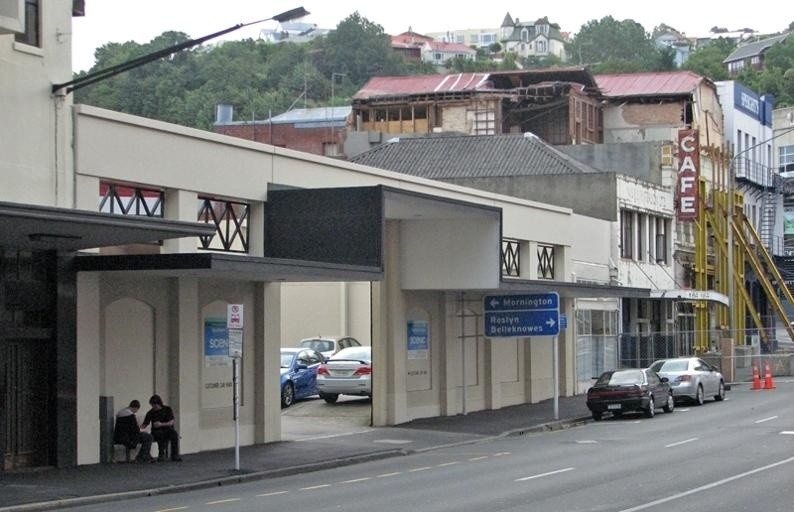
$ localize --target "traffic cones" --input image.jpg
[762,360,776,389]
[750,360,763,390]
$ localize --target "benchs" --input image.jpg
[113,433,182,464]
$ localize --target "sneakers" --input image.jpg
[157,456,164,462]
[171,455,182,462]
[135,454,155,464]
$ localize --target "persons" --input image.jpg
[138,393,183,462]
[113,399,155,463]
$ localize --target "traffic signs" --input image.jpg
[481,292,561,338]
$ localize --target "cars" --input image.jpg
[298,336,362,360]
[314,345,371,403]
[585,368,674,421]
[648,358,726,405]
[280,347,327,408]
